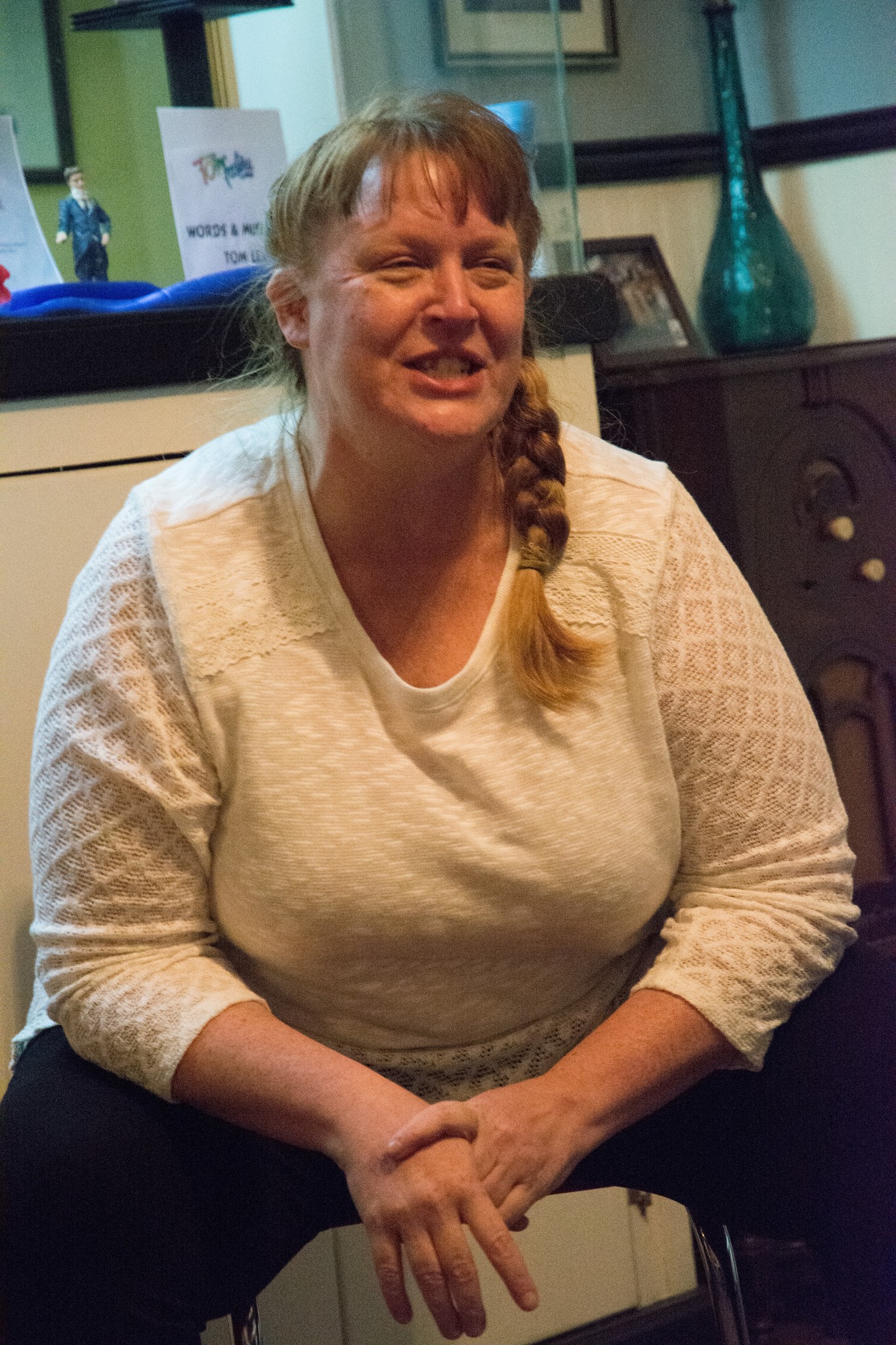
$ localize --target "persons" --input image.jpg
[52,166,121,285]
[0,92,896,1345]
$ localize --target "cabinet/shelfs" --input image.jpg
[1,348,640,1078]
[593,334,896,903]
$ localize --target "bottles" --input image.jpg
[481,97,560,276]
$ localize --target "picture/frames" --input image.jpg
[433,0,620,68]
[549,238,709,366]
[1,0,78,186]
